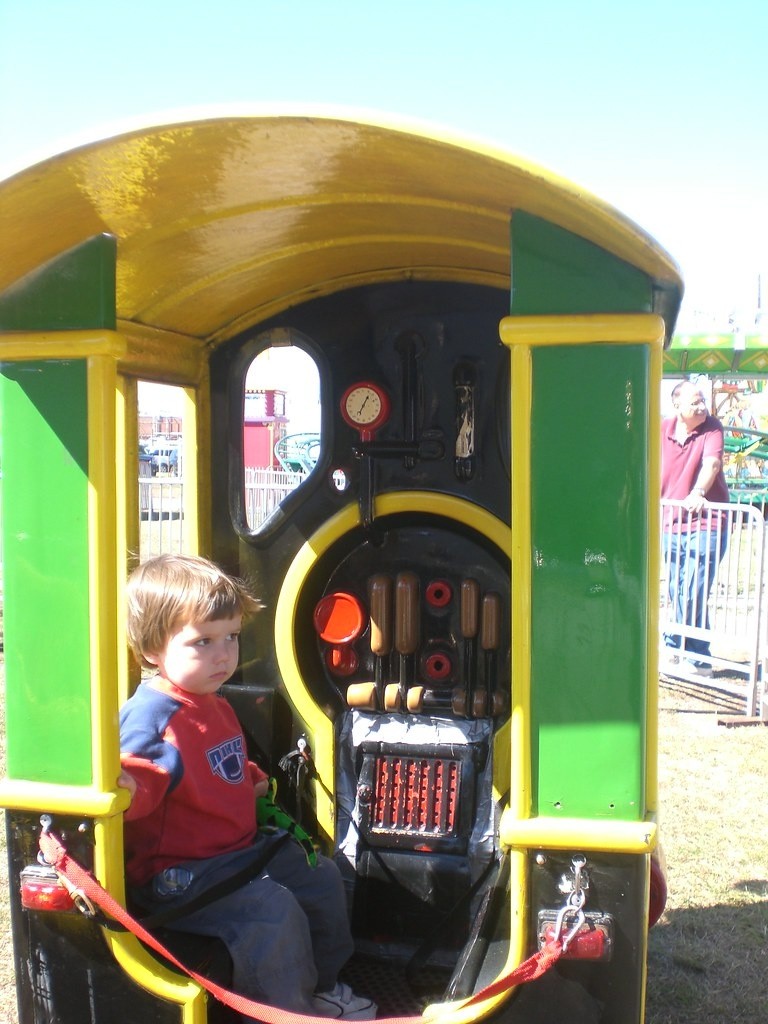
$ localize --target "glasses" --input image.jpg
[678,398,707,407]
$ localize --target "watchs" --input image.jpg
[690,488,706,497]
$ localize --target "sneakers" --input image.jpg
[311,980,384,1021]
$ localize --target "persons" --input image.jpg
[113,553,380,1019]
[660,378,727,676]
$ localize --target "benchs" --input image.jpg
[8,685,291,1024]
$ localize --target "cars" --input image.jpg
[139,434,180,474]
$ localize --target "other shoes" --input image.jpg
[672,660,713,676]
[662,653,685,668]
